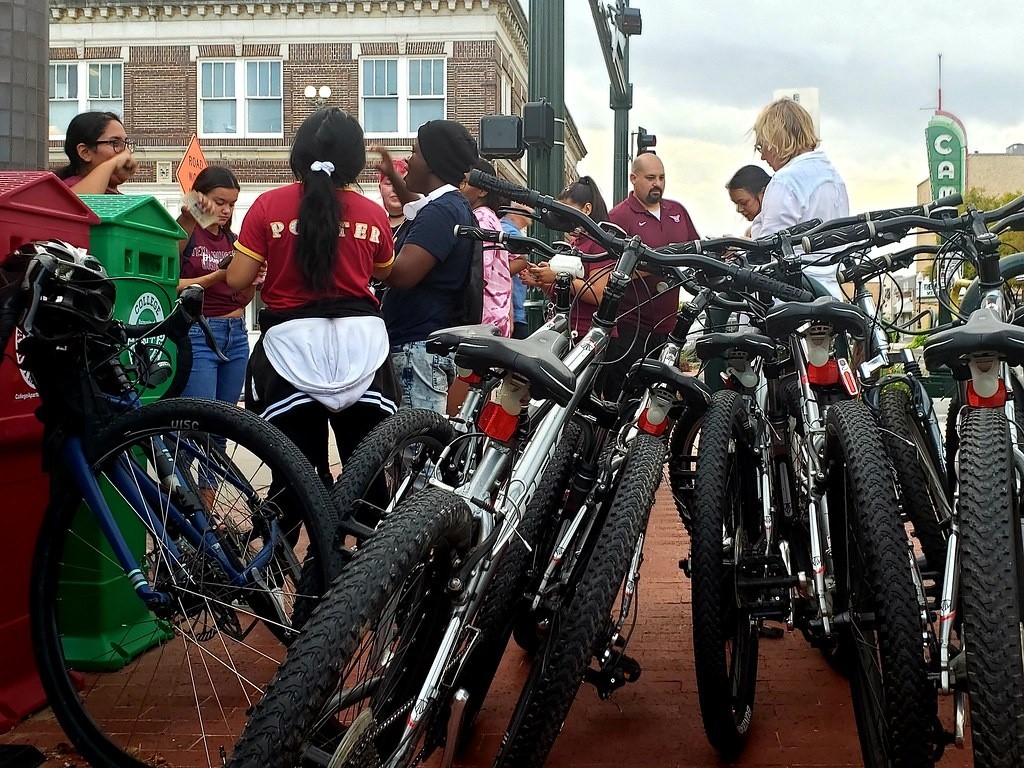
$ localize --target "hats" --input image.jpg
[418,120,479,188]
[379,157,408,181]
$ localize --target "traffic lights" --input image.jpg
[637,126,656,157]
[622,3,642,38]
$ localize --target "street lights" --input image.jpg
[916,271,923,329]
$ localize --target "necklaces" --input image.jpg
[389,213,404,218]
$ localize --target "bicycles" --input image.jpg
[0,168,1024,768]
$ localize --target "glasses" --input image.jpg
[754,140,764,151]
[92,139,136,154]
[459,180,468,189]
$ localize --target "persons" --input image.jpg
[501,201,536,340]
[723,165,772,259]
[225,106,403,613]
[460,158,511,338]
[51,110,215,255]
[750,100,850,307]
[367,157,408,299]
[519,175,618,400]
[609,153,700,375]
[163,165,256,529]
[369,120,480,490]
